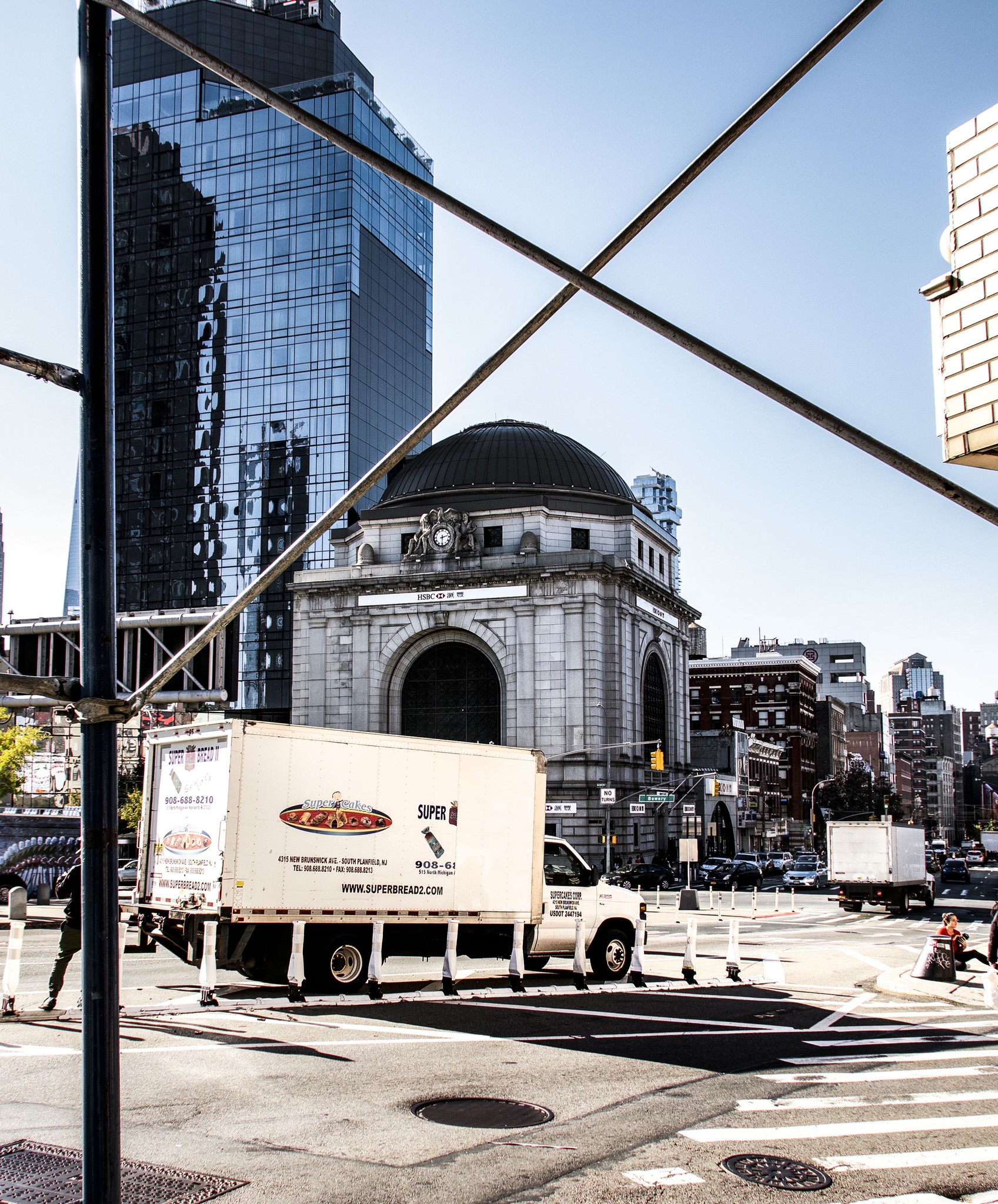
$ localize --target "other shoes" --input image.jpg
[38,997,56,1010]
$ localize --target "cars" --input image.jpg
[753,852,775,876]
[698,857,732,881]
[925,838,987,884]
[702,861,763,891]
[793,851,818,860]
[733,852,763,870]
[782,861,830,890]
[600,863,675,891]
[117,859,138,886]
[788,855,828,868]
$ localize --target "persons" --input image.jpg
[601,853,672,873]
[935,912,998,971]
[938,852,947,867]
[987,902,998,968]
[41,850,107,1010]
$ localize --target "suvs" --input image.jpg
[768,851,793,874]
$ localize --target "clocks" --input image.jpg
[428,522,455,552]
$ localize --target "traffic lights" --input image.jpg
[650,751,660,771]
[884,795,889,810]
[712,781,720,796]
[612,836,618,844]
[597,836,605,844]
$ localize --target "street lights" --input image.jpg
[762,746,793,831]
[597,702,610,874]
[812,778,836,850]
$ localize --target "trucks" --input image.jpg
[116,716,648,995]
[980,831,998,863]
[825,821,936,916]
